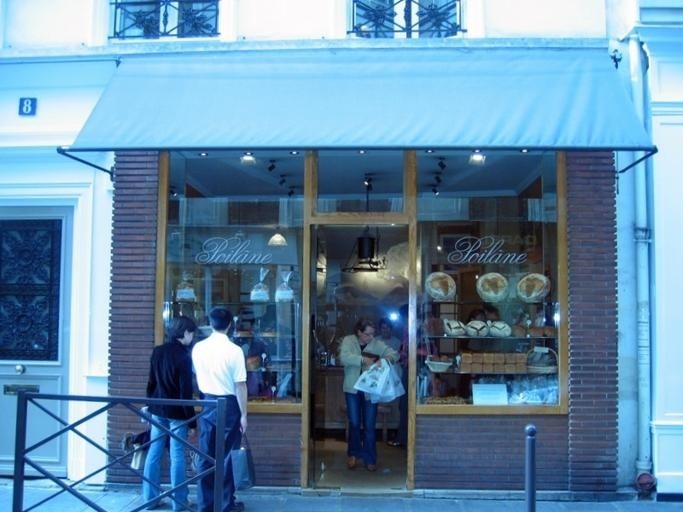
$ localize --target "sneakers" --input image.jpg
[388,439,406,448]
[348,455,376,472]
[143,496,243,512]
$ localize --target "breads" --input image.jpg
[443,320,558,338]
[460,353,528,374]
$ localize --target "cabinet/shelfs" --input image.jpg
[162,299,303,404]
[419,302,560,408]
[364,177,373,192]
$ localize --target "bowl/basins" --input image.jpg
[198,325,213,337]
[423,360,452,373]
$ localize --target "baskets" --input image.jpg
[425,352,452,373]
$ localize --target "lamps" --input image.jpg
[432,157,446,196]
[268,234,287,246]
[342,226,388,273]
[268,160,295,197]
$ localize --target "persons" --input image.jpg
[229,307,271,400]
[374,318,401,351]
[466,306,500,399]
[192,306,248,512]
[146,316,196,512]
[340,318,401,471]
[387,303,438,446]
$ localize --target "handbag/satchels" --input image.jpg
[231,428,256,490]
[354,359,405,405]
[129,429,149,471]
